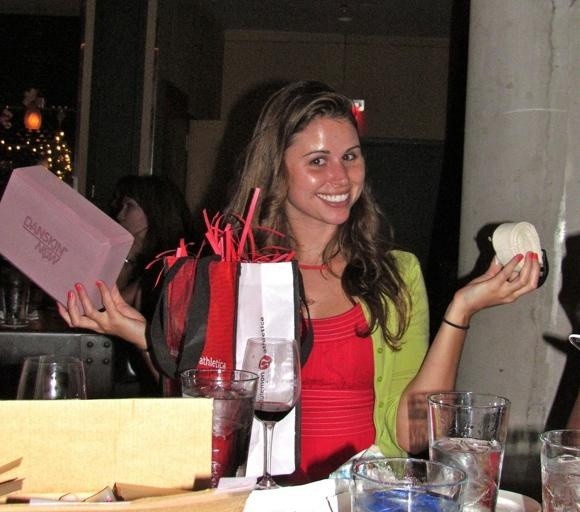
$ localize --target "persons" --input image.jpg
[0,174,195,400]
[54,82,544,489]
[560,394,580,458]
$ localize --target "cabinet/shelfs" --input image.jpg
[219,31,450,142]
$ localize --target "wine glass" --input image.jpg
[240,336,302,490]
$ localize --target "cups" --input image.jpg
[537,428,580,512]
[349,457,467,512]
[180,367,260,489]
[16,354,88,400]
[2,272,33,326]
[427,390,510,512]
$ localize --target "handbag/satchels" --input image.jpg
[156,257,301,478]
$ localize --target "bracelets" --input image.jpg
[442,316,470,330]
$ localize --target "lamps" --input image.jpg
[24,100,42,130]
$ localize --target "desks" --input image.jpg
[0,319,115,399]
